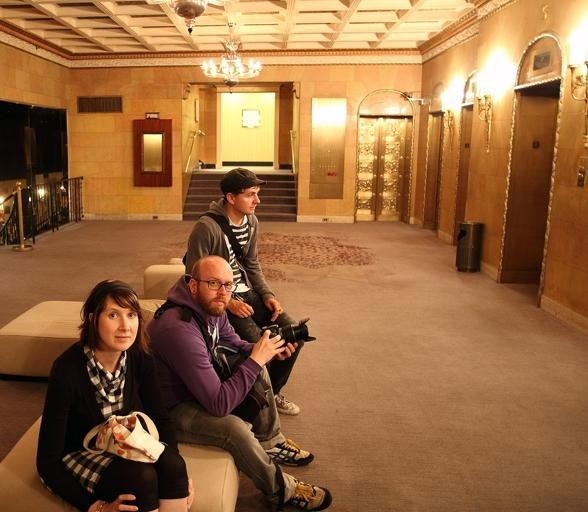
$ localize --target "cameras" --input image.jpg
[260,317,316,348]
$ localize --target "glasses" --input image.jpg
[192,277,238,291]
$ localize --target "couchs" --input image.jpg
[0,300,242,511]
[144,257,186,300]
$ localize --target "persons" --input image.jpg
[36,279,193,512]
[185,168,305,415]
[144,255,332,512]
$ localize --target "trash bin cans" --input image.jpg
[455,222,485,275]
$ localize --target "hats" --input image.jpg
[220,168,265,194]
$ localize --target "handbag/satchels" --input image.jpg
[83,412,165,463]
[153,299,270,417]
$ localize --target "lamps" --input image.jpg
[568,59,588,187]
[200,49,263,93]
[476,94,493,155]
[145,0,228,36]
[241,109,261,129]
[447,108,453,148]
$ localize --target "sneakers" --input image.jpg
[264,439,314,466]
[281,471,332,512]
[275,393,301,416]
